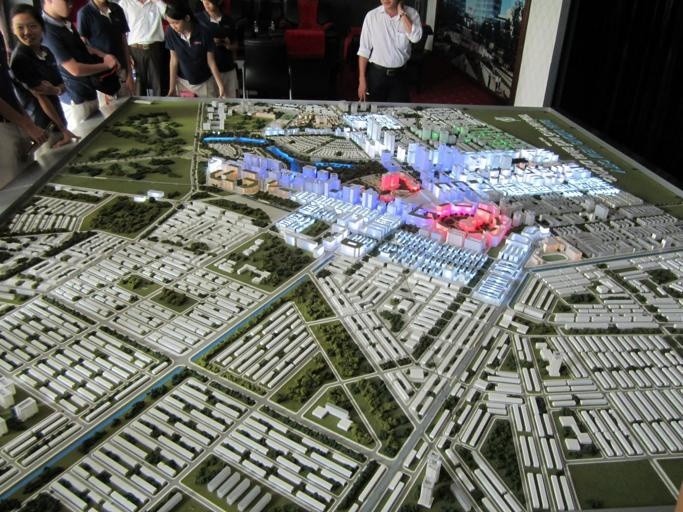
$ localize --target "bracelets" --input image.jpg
[398,11,408,19]
[56,86,62,96]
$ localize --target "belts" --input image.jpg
[371,62,408,75]
[130,42,163,50]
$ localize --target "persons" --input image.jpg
[193,0,238,98]
[75,0,134,109]
[118,0,172,96]
[40,1,120,133]
[354,1,422,103]
[5,3,81,149]
[162,4,227,98]
[0,32,49,191]
